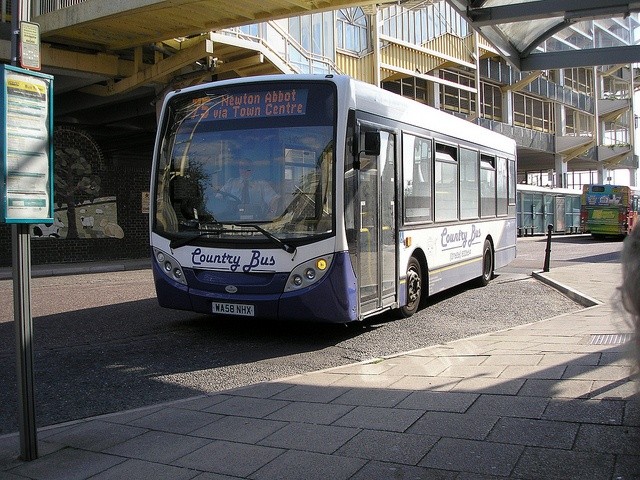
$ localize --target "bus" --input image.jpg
[149,74,517,323]
[580,184,640,239]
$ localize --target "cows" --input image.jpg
[99,218,124,239]
[32,217,65,239]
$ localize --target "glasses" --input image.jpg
[240,166,249,170]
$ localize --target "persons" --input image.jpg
[216,157,279,211]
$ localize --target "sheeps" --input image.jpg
[81,215,94,229]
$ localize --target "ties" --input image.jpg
[242,183,250,205]
